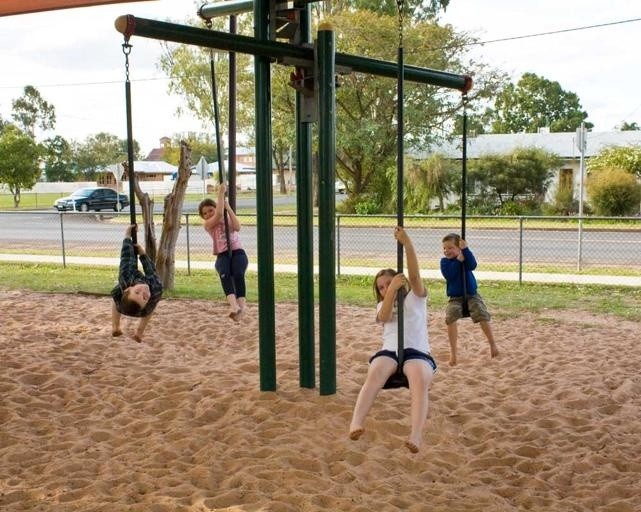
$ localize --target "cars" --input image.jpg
[54,187,130,212]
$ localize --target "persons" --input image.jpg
[198,181,250,321]
[110,222,165,345]
[439,233,500,367]
[347,226,437,453]
